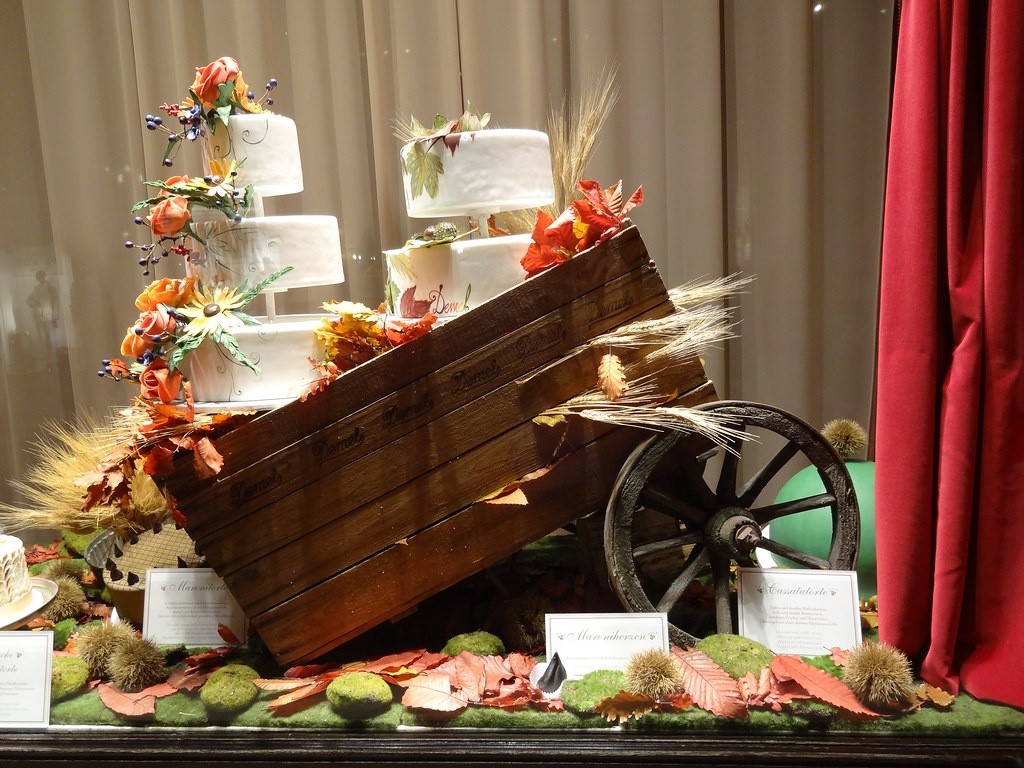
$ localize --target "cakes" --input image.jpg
[182,215,345,290]
[399,129,556,219]
[384,232,533,320]
[201,115,303,196]
[0,534,32,608]
[172,314,342,401]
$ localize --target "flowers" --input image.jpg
[121,58,263,406]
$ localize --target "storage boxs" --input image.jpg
[147,224,723,666]
